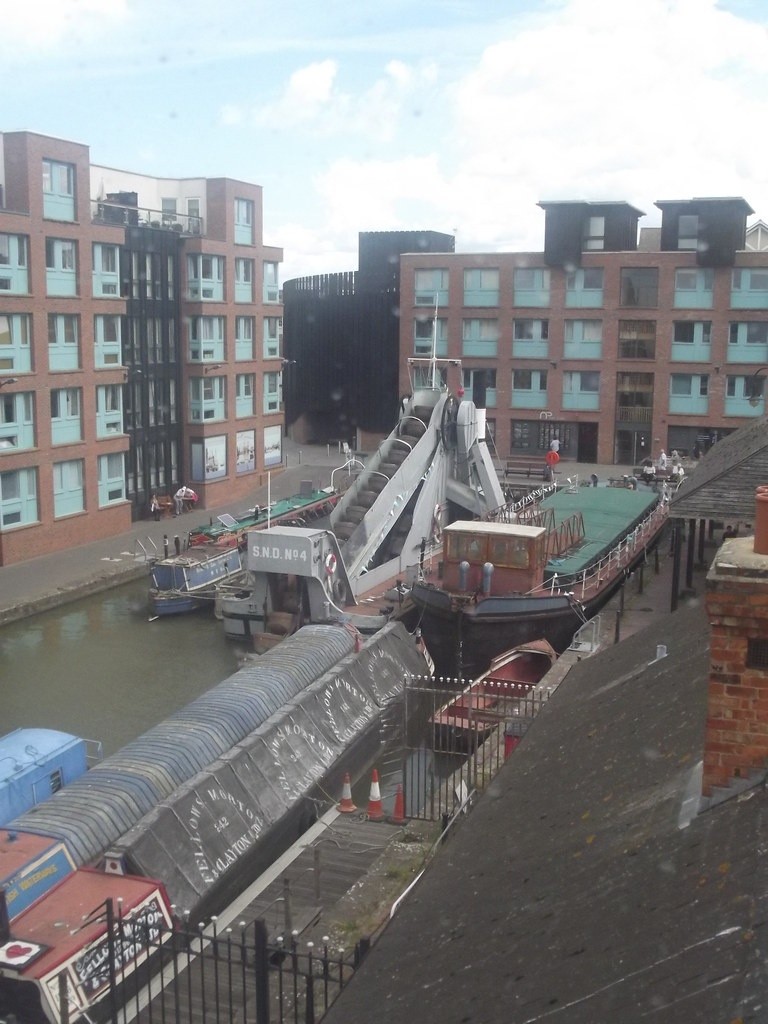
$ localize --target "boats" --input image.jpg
[409,473,671,641]
[428,634,557,746]
[0,622,436,1023]
[1,724,107,830]
[150,472,358,638]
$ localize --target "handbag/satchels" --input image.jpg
[191,493,198,502]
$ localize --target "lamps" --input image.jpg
[550,361,557,368]
[205,365,222,373]
[0,379,18,388]
[124,370,141,379]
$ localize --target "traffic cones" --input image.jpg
[387,784,408,824]
[336,773,358,813]
[360,768,387,823]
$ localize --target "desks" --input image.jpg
[160,502,173,518]
[607,477,633,488]
[182,498,195,512]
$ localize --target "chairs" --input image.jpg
[157,495,173,515]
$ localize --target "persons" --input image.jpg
[550,436,702,488]
[173,486,198,516]
[254,505,259,520]
[722,526,736,541]
[150,494,161,521]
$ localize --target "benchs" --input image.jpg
[504,461,546,477]
[632,469,672,486]
